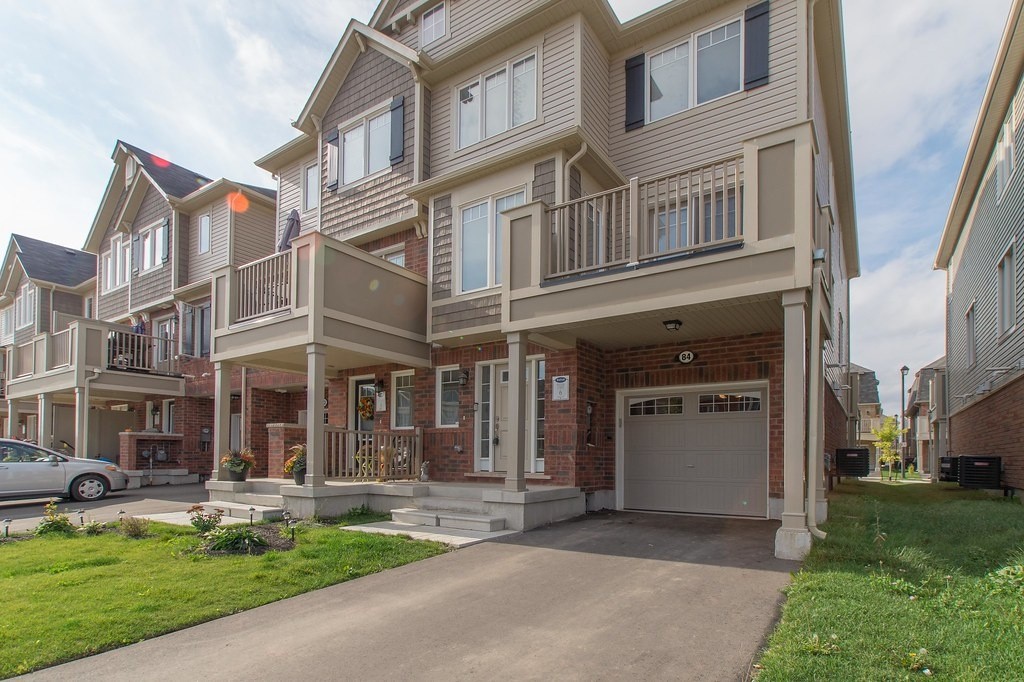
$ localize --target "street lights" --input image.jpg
[901,366,909,477]
[895,414,898,454]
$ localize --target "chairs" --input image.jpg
[389,436,416,474]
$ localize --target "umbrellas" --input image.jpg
[278,209,300,252]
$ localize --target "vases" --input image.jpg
[293,470,305,485]
[229,466,247,481]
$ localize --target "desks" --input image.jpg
[351,456,379,482]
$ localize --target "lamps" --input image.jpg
[831,381,852,391]
[975,380,991,397]
[373,377,385,397]
[662,319,683,332]
[459,369,469,386]
[151,407,159,416]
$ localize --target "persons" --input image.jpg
[913,456,916,472]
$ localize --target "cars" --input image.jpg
[895,456,918,471]
[0,437,129,502]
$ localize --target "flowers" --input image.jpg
[358,398,374,419]
[219,447,257,473]
[284,444,307,473]
